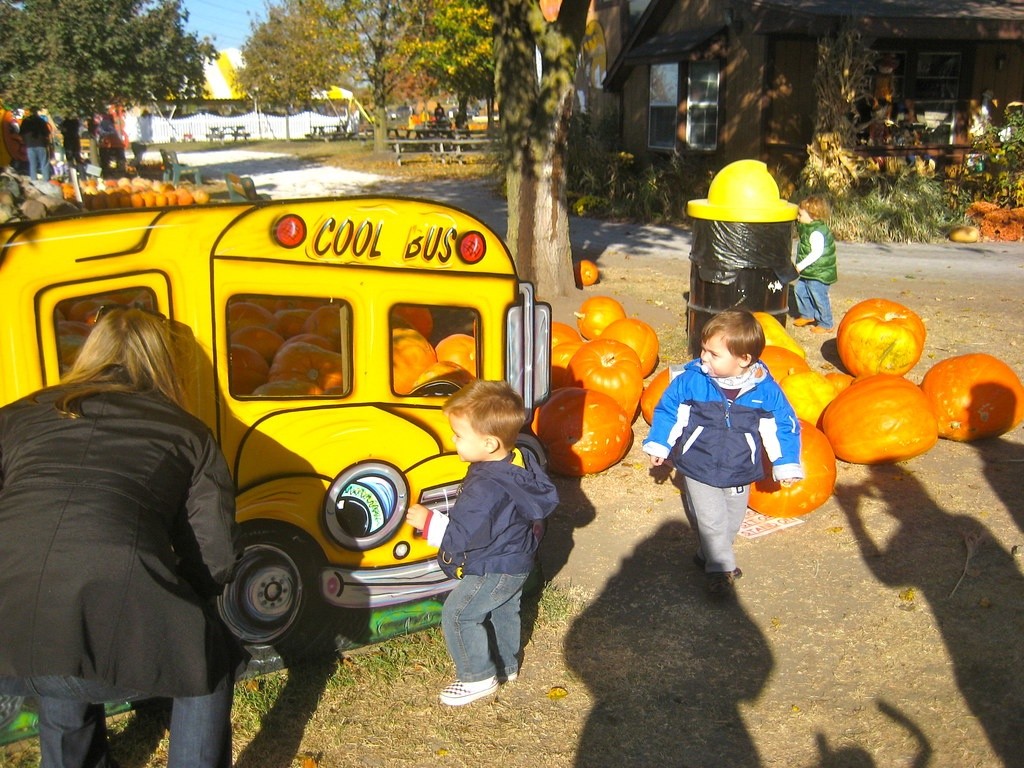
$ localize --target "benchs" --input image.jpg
[206,132,250,144]
[224,172,273,203]
[305,132,356,142]
[367,135,502,156]
[160,148,202,186]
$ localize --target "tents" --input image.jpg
[124,46,356,146]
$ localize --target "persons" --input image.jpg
[19,105,50,183]
[434,103,444,124]
[405,379,561,707]
[59,114,88,182]
[96,115,127,178]
[792,198,837,335]
[0,303,243,768]
[644,308,801,596]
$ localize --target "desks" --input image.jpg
[456,129,490,137]
[384,139,449,167]
[451,140,500,166]
[311,125,348,134]
[365,128,399,138]
[406,128,454,139]
[211,125,245,134]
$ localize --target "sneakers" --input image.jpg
[497,672,517,681]
[440,674,500,706]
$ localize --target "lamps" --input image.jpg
[995,54,1006,70]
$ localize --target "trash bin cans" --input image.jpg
[687,158,797,363]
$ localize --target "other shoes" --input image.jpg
[794,318,814,328]
[710,571,734,592]
[810,324,834,336]
[694,553,743,579]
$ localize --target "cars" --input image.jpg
[386,105,413,123]
[447,106,480,120]
[428,113,452,128]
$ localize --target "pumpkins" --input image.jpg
[949,226,978,242]
[523,297,1024,518]
[573,260,598,286]
[0,173,476,398]
